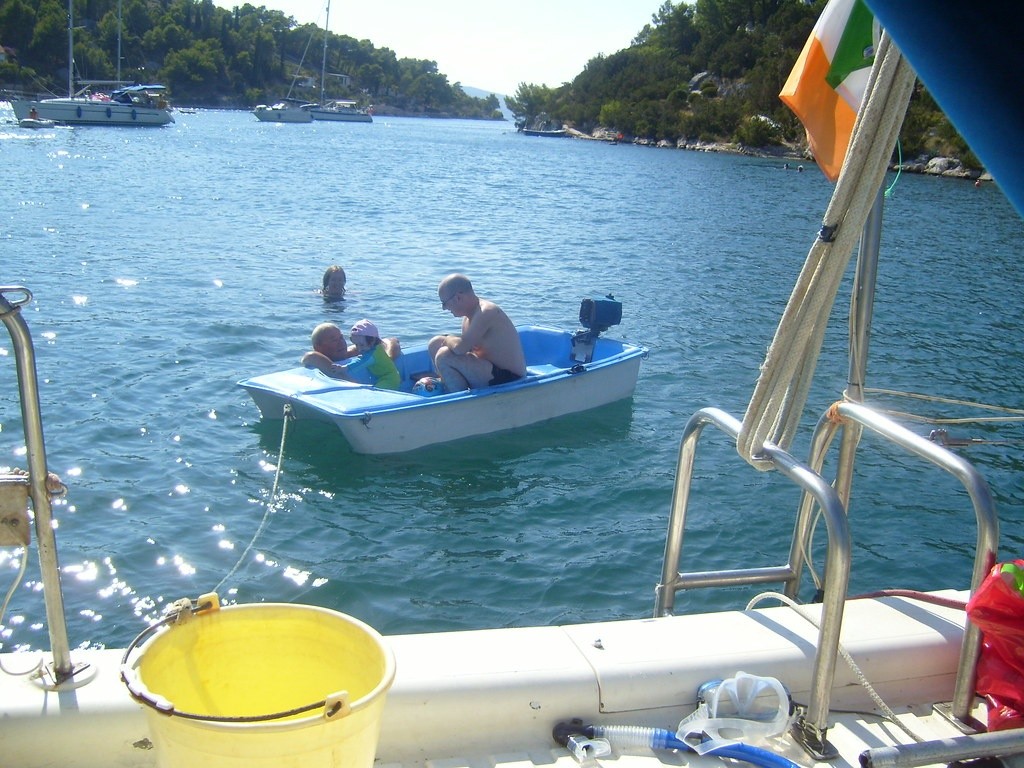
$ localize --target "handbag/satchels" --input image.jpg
[964,559,1024,732]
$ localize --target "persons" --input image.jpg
[796,165,804,172]
[783,163,790,170]
[429,273,528,393]
[331,319,401,391]
[613,131,623,142]
[301,322,399,380]
[323,265,346,296]
[30,105,39,120]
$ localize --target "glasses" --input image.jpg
[441,292,462,305]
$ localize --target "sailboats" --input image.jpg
[11,0,175,128]
[285,0,374,122]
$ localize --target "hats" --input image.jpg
[351,320,384,345]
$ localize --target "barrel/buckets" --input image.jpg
[119,593,395,768]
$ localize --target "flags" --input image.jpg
[779,0,883,186]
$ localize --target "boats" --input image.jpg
[522,129,566,138]
[609,142,618,145]
[19,119,53,129]
[251,103,313,123]
[180,110,195,113]
[237,297,653,455]
